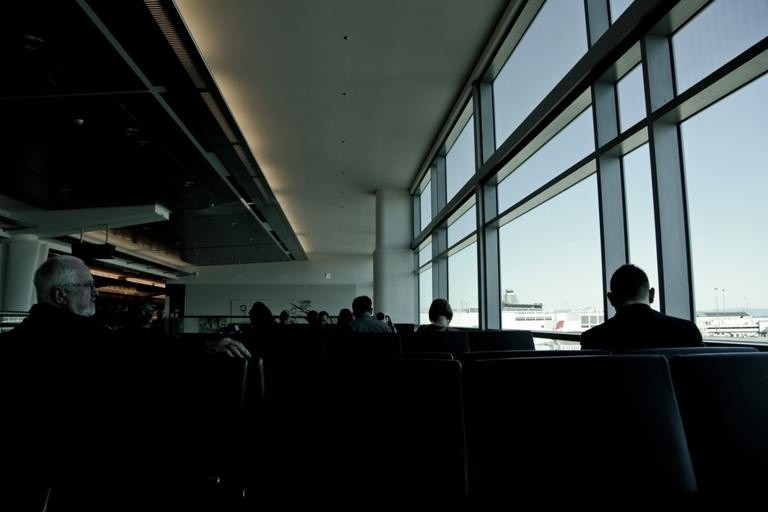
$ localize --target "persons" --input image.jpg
[2,251,456,361]
[577,263,706,350]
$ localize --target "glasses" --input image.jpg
[60,282,96,292]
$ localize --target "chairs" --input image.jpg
[0,323,768,512]
